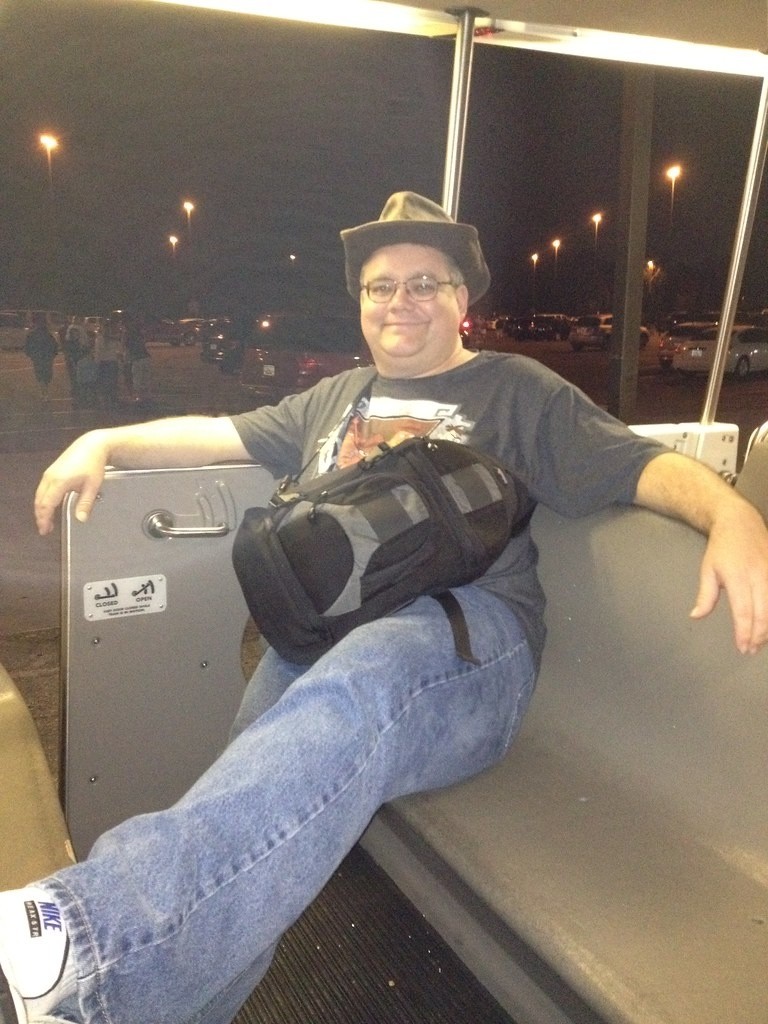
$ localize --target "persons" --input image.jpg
[0,191,768,1024]
[495,319,504,341]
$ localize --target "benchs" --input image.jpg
[357,501,767,1024]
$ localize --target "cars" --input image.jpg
[0,309,240,370]
[511,314,567,339]
[569,314,649,351]
[658,322,717,369]
[672,325,768,381]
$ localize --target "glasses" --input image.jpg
[359,274,459,303]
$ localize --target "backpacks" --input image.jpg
[231,437,536,667]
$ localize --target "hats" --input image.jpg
[339,191,491,308]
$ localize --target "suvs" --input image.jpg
[239,312,375,402]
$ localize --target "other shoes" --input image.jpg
[0,886,71,1024]
